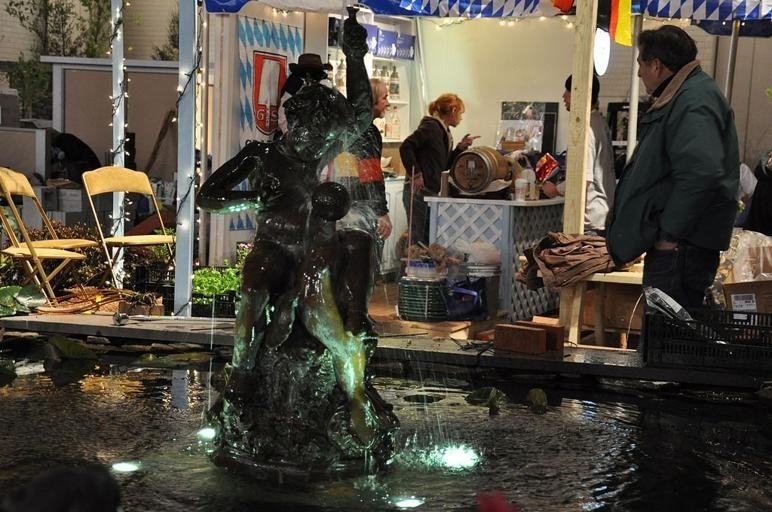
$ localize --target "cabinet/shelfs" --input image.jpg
[327,14,415,283]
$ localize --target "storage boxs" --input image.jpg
[722,280,772,339]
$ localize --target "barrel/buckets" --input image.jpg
[452,146,506,193]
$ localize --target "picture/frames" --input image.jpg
[608,101,652,180]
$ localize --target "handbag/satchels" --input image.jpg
[448,276,488,321]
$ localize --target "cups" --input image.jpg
[515,179,527,202]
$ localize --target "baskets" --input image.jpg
[643,305,772,371]
[136,262,236,318]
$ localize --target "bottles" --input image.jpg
[378,105,401,139]
[336,59,346,88]
[373,65,399,94]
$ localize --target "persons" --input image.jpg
[195,42,378,448]
[604,25,739,310]
[400,92,482,246]
[542,73,617,236]
[326,78,393,324]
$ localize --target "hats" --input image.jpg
[289,54,333,72]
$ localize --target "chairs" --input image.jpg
[0,166,176,308]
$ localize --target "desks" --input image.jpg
[519,257,644,346]
